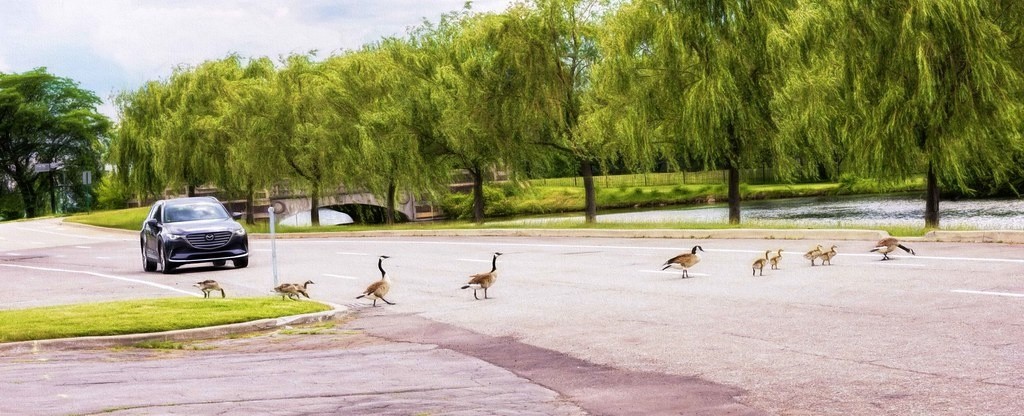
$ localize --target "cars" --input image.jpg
[139,196,248,274]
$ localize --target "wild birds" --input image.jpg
[274,279,314,301]
[869,237,915,260]
[461,252,504,300]
[751,250,772,276]
[769,249,785,270]
[660,246,704,278]
[820,245,838,265]
[802,244,824,266]
[192,279,225,300]
[356,256,396,307]
